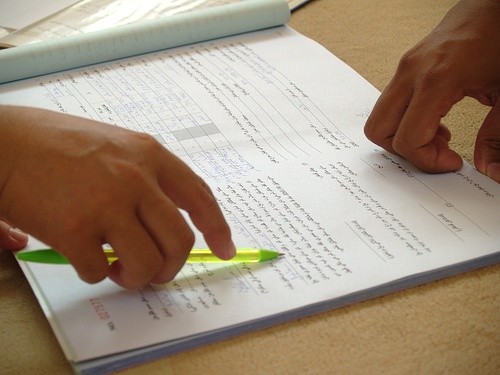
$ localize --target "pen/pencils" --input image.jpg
[15,247,286,265]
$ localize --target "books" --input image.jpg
[0,0,500,375]
[0,0,309,49]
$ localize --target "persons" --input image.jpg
[364,0,500,182]
[0,104,237,294]
